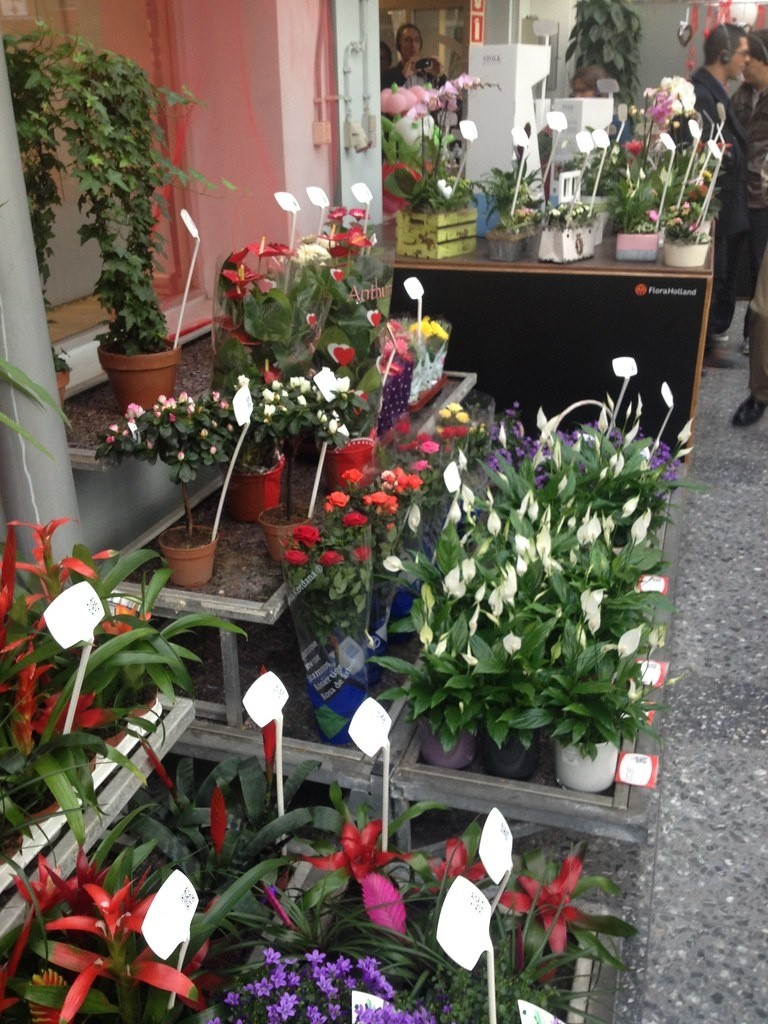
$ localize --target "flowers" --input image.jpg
[0,71,734,1023]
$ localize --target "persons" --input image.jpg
[379,25,463,150]
[665,19,767,426]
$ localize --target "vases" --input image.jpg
[95,336,182,412]
[484,724,541,781]
[219,452,286,523]
[394,207,477,258]
[663,239,709,267]
[409,373,446,415]
[555,735,617,792]
[592,218,604,246]
[582,196,611,230]
[54,368,73,406]
[615,231,657,261]
[418,713,476,767]
[485,229,530,261]
[256,503,314,561]
[538,223,594,263]
[158,524,221,589]
[322,442,375,492]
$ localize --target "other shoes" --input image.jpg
[732,394,768,426]
[739,336,751,354]
[711,332,729,341]
